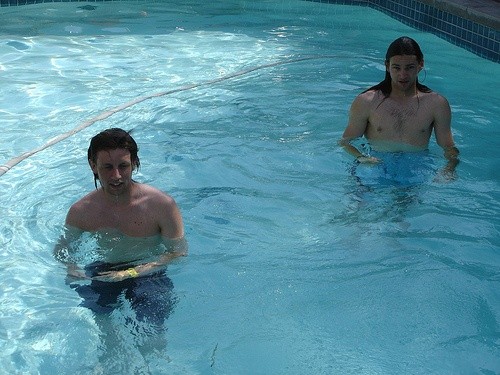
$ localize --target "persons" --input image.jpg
[338,36,459,223]
[55,127,187,372]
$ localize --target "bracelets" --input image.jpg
[127,268,138,278]
